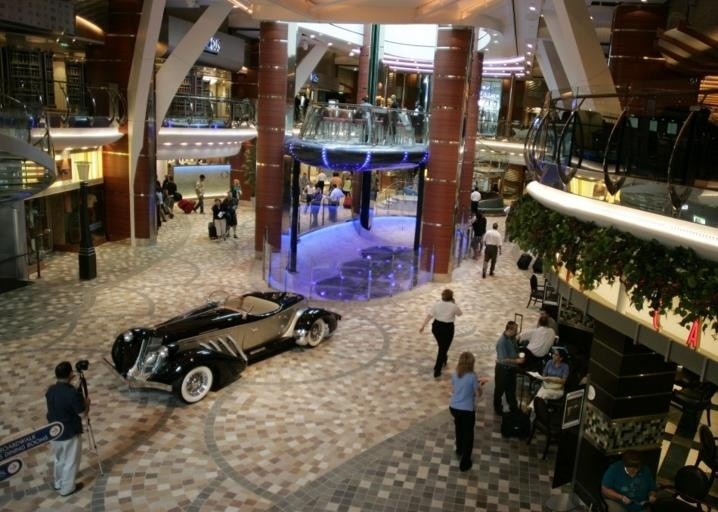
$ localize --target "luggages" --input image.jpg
[209,215,216,238]
[516,253,532,271]
[501,374,533,440]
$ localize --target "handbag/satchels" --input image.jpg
[174,192,182,201]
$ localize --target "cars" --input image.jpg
[402,184,417,196]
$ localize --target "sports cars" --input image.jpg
[101,291,342,405]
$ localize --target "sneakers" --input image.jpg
[62,482,84,496]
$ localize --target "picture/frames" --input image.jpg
[560,389,584,430]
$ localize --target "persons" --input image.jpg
[299,168,352,227]
[294,91,424,144]
[447,352,489,474]
[419,290,462,380]
[494,308,572,417]
[42,359,89,498]
[600,452,657,512]
[152,170,243,243]
[469,187,501,280]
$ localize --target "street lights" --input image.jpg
[74,160,97,280]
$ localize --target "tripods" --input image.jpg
[77,378,105,476]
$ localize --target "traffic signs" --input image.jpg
[0,458,24,483]
[0,421,64,462]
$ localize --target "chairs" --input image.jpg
[527,274,544,310]
[527,395,562,460]
[316,107,411,146]
[593,377,717,511]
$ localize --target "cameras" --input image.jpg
[76,360,89,373]
